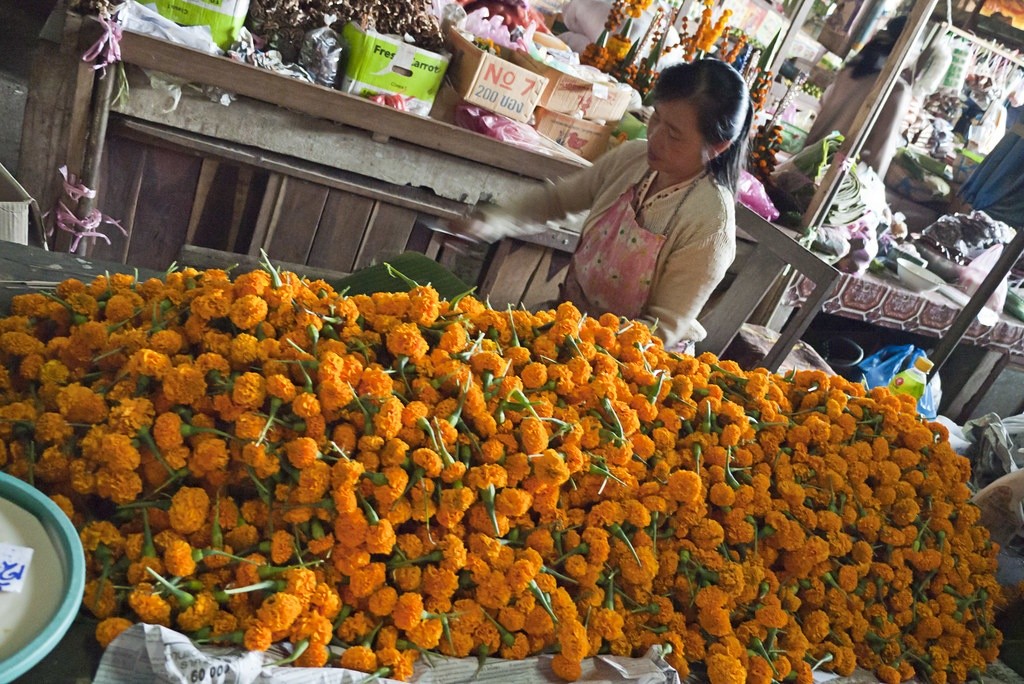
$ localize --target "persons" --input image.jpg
[445,57,756,359]
[800,16,943,182]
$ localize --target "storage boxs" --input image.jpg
[440,18,549,125]
[133,0,251,57]
[529,105,614,164]
[609,110,649,142]
[516,47,634,128]
[339,19,453,120]
[0,161,50,253]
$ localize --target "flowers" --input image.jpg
[0,246,1007,684]
